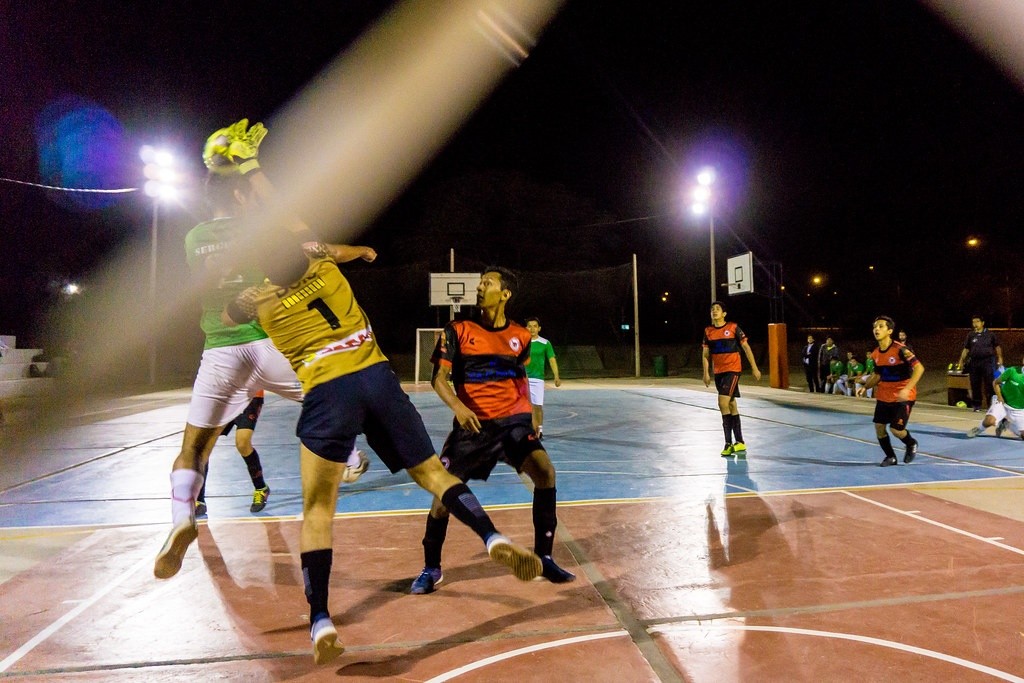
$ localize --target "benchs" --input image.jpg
[0,335,57,398]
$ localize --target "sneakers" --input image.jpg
[541,556,576,584]
[195,501,207,515]
[484,532,543,581]
[411,567,444,593]
[996,418,1006,437]
[155,519,199,578]
[250,483,270,513]
[904,439,918,463]
[880,455,898,467]
[966,424,985,437]
[721,442,734,455]
[310,612,345,665]
[341,452,369,483]
[733,441,746,451]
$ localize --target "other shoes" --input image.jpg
[538,426,543,439]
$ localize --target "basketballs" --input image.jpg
[200,129,243,174]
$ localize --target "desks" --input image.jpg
[947,374,988,409]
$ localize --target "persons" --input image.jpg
[818,335,839,393]
[155,118,369,578]
[855,317,924,467]
[221,226,543,665]
[898,329,916,357]
[801,334,820,392]
[411,266,576,595]
[520,316,561,440]
[959,315,1003,413]
[825,350,874,398]
[966,357,1024,441]
[702,301,761,456]
